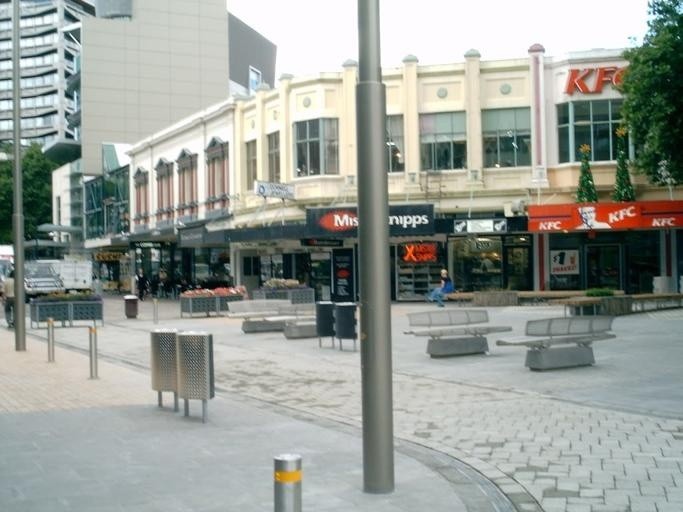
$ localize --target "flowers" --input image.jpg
[179,284,248,298]
[29,287,102,304]
[260,278,307,294]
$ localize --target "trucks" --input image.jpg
[0,245,15,300]
[35,259,92,295]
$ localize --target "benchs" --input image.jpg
[445,290,683,318]
[403,310,616,371]
[225,299,317,339]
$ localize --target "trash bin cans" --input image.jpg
[334,302,357,351]
[150,329,180,412]
[177,331,214,423]
[123,295,138,319]
[315,301,336,348]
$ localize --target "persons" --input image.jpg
[426,269,453,307]
[2,270,16,328]
[134,268,186,301]
[574,207,612,229]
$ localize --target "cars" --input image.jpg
[23,262,66,303]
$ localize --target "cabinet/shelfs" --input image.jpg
[395,242,444,302]
